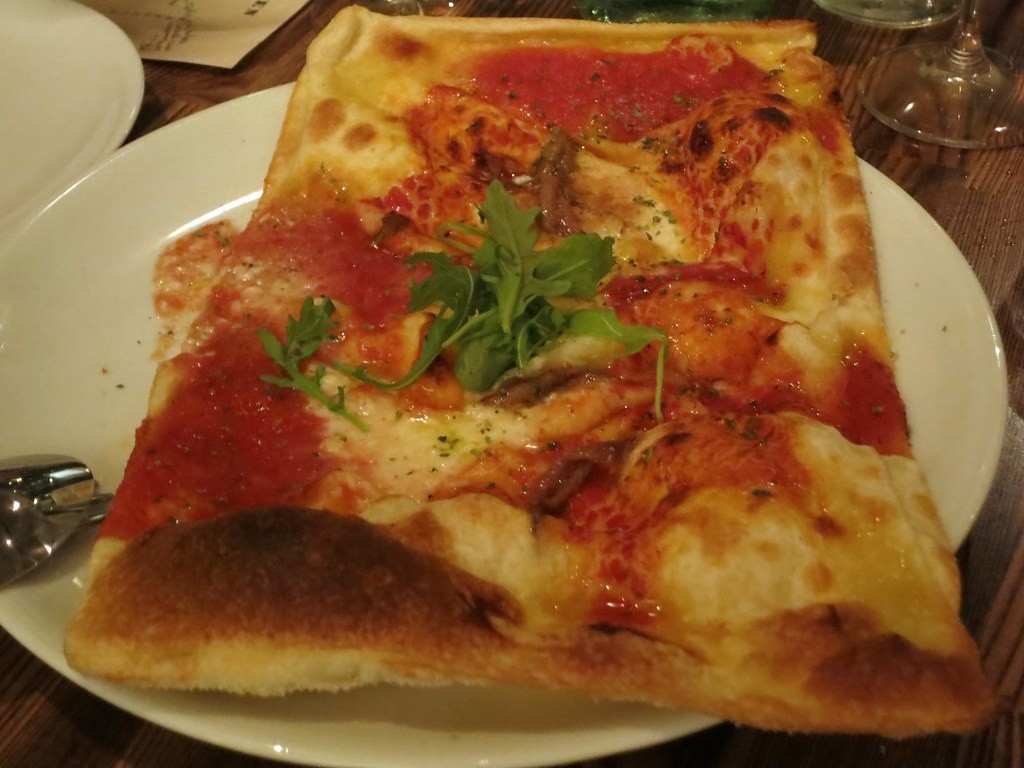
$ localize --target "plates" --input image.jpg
[0,0,146,231]
[0,82,1009,768]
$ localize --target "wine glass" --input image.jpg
[858,0,1024,149]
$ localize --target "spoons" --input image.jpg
[0,454,95,590]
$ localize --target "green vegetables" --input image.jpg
[245,172,672,423]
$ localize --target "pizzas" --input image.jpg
[61,0,1001,741]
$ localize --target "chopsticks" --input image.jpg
[77,493,113,527]
